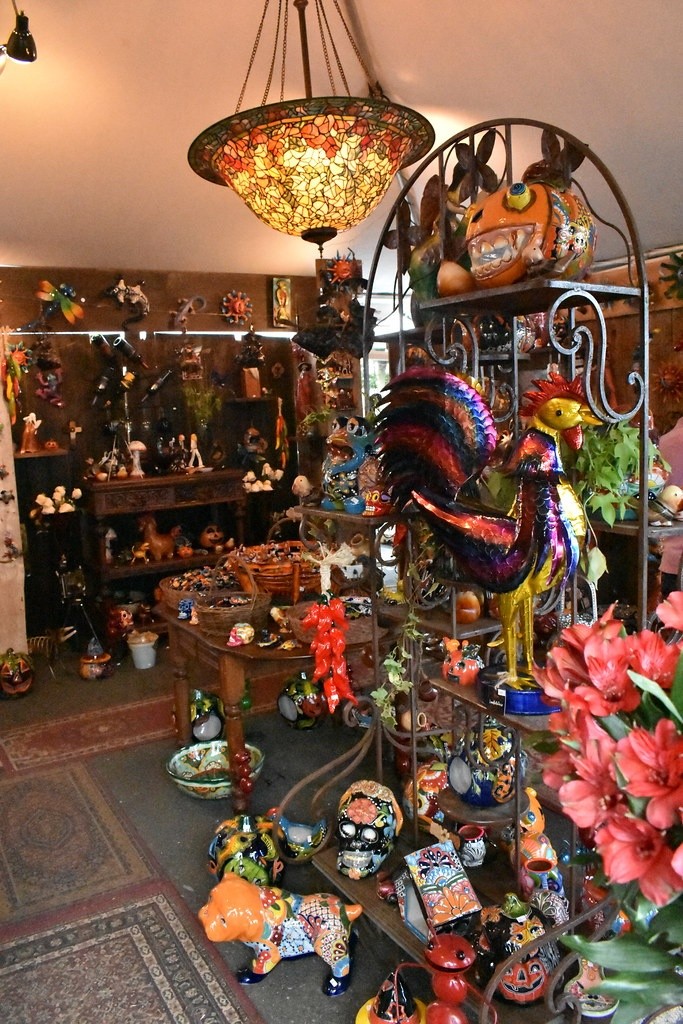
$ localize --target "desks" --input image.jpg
[153,598,395,816]
[588,520,683,596]
[380,592,504,666]
[78,470,246,582]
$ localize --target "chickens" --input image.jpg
[372,366,603,694]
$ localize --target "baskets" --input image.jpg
[193,553,272,638]
[159,571,233,611]
[287,600,378,644]
[228,517,340,592]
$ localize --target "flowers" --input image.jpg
[546,591,683,1024]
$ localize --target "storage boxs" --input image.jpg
[240,368,260,398]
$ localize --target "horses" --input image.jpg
[137,513,182,565]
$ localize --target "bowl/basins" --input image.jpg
[165,739,266,799]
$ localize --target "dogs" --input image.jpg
[198,872,364,997]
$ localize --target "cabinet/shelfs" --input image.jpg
[295,115,683,1024]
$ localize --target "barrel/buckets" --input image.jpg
[129,641,155,670]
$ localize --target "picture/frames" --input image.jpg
[273,276,298,329]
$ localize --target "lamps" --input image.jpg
[189,0,434,254]
[0,0,39,64]
